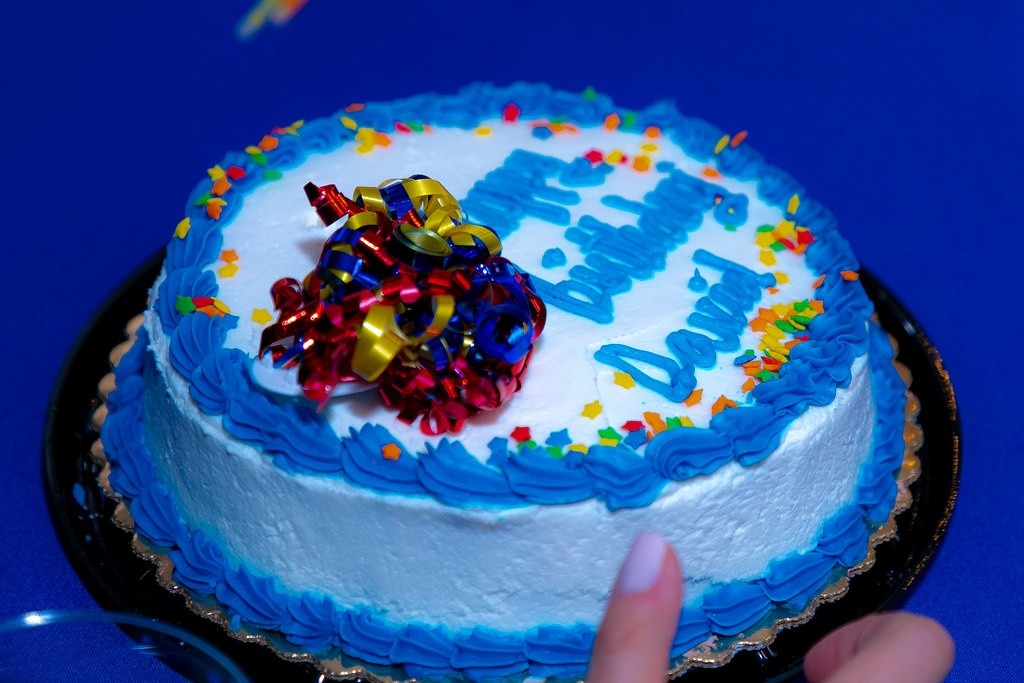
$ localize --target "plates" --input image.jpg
[42,241,959,683]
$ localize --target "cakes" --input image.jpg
[89,78,926,683]
